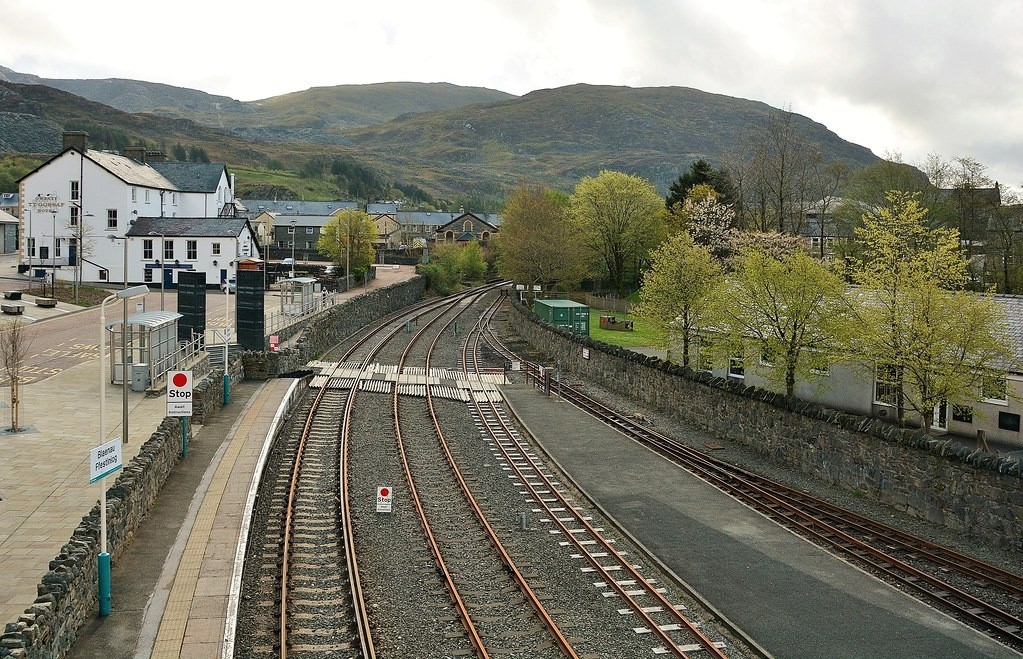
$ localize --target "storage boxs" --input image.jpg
[535,300,589,338]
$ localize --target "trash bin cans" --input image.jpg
[314,283,321,293]
[600,315,615,329]
[131,363,149,392]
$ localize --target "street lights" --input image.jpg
[345,206,351,291]
[290,219,296,302]
[51,210,56,296]
[262,221,266,289]
[23,208,32,293]
[148,231,165,309]
[107,234,128,444]
[98,284,150,614]
[73,214,96,302]
[228,229,239,270]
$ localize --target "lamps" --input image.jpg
[175,260,179,264]
[155,259,160,264]
[230,262,233,266]
[213,260,218,266]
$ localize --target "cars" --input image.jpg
[282,258,296,265]
[219,279,236,294]
[399,244,407,250]
[276,266,343,291]
